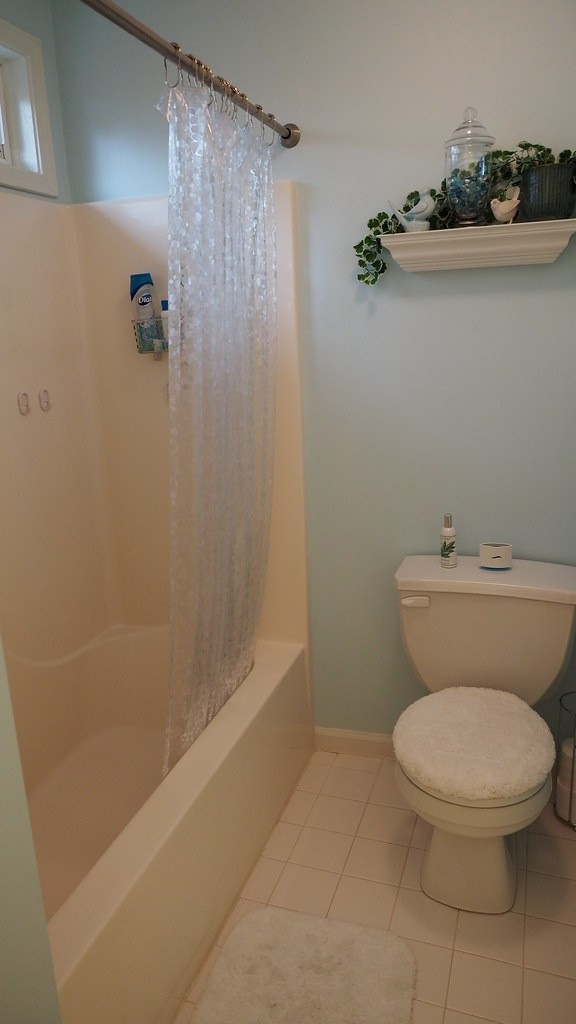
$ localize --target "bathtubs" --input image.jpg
[0,624,314,1024]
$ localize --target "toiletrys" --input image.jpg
[158,297,172,351]
[439,512,459,571]
[127,270,163,351]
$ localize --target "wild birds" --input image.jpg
[402,186,436,221]
[490,199,521,224]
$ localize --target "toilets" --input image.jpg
[391,553,576,916]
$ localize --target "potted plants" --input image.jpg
[348,139,576,286]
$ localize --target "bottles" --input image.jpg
[445,107,495,228]
[439,513,457,570]
